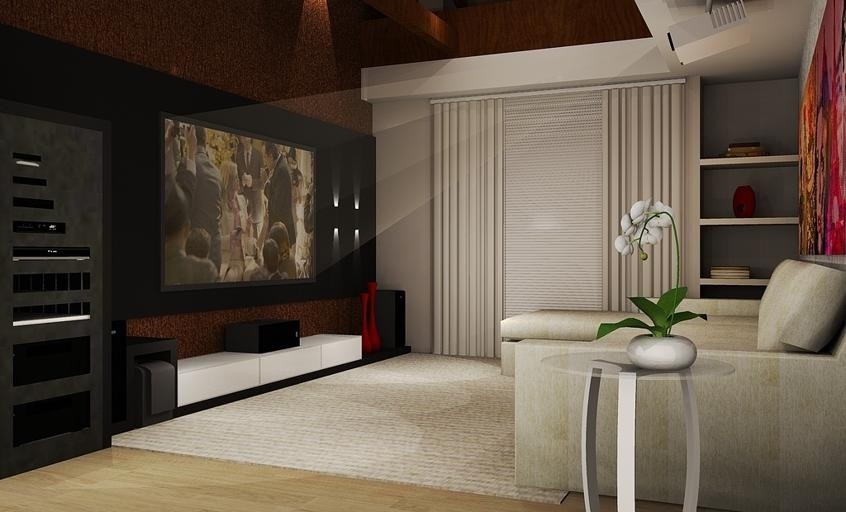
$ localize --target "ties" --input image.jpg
[247,151,249,168]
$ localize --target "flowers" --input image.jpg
[595,198,709,341]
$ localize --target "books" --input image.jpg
[710,266,753,279]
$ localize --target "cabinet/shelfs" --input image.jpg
[698,153,801,288]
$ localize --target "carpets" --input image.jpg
[110,349,569,505]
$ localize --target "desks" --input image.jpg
[543,351,734,512]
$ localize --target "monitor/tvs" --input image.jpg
[158,111,317,293]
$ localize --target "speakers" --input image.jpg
[371,289,406,349]
[110,319,128,425]
[223,319,300,354]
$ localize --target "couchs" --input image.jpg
[500,256,845,512]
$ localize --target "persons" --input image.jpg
[164,119,314,284]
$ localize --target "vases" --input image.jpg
[625,335,697,371]
